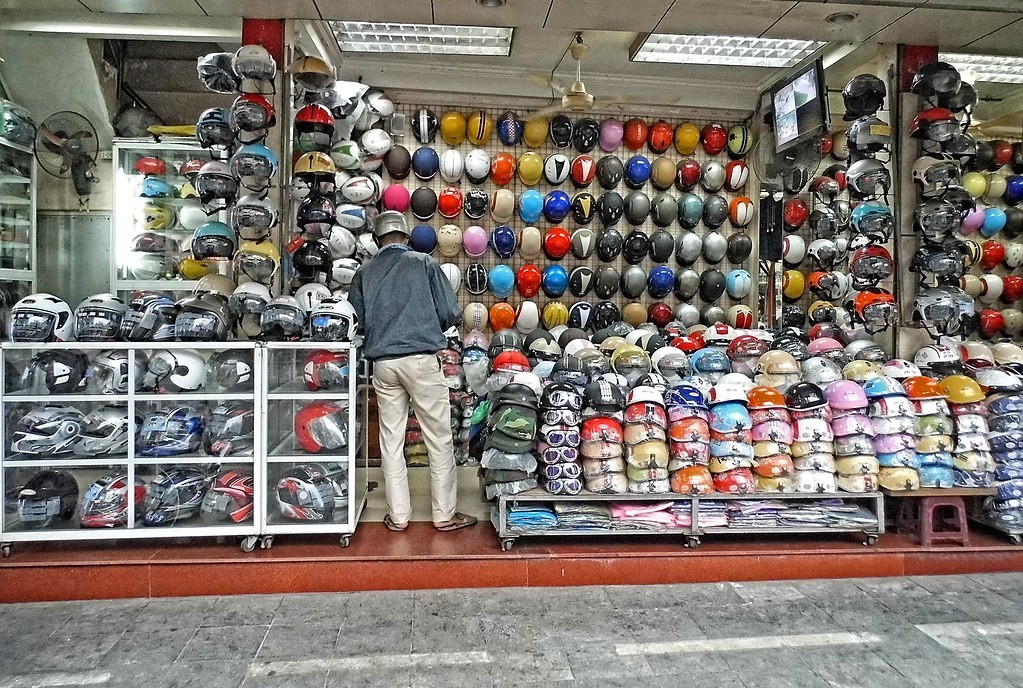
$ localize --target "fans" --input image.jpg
[753,126,823,193]
[514,44,681,122]
[34,110,100,196]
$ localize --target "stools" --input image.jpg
[894,496,972,548]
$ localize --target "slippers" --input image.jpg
[438,512,477,532]
[383,513,404,531]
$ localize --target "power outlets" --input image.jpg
[101,151,112,159]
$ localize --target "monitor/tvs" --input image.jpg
[770,58,830,153]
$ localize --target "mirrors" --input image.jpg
[757,183,784,331]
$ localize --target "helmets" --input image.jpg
[383,184,409,214]
[118,44,394,301]
[409,61,1023,497]
[985,497,1023,526]
[384,143,411,180]
[2,104,36,147]
[7,290,366,526]
[372,209,410,244]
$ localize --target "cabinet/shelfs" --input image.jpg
[0,335,369,559]
[109,136,234,308]
[0,135,37,296]
[489,479,885,550]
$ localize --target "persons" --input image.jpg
[345,212,479,532]
